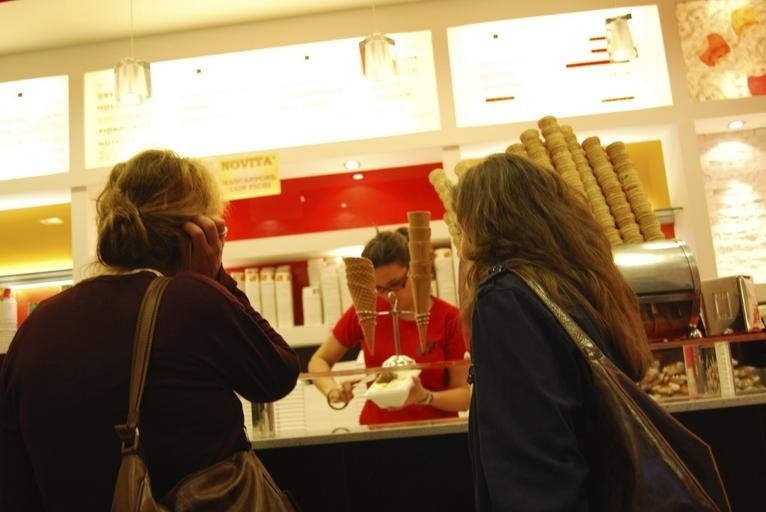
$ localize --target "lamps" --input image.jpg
[358,0,397,79]
[605,0,639,65]
[114,0,150,108]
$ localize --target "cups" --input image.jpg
[0,288,21,353]
[428,250,456,312]
[229,249,354,328]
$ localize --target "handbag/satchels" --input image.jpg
[112,275,294,512]
[475,262,733,512]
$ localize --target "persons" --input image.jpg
[0,150,300,511]
[452,153,652,511]
[307,227,471,425]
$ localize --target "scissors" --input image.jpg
[327,376,374,410]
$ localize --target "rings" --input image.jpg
[219,226,228,235]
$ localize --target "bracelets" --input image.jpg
[327,389,348,410]
[415,391,432,405]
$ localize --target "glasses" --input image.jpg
[376,264,409,293]
